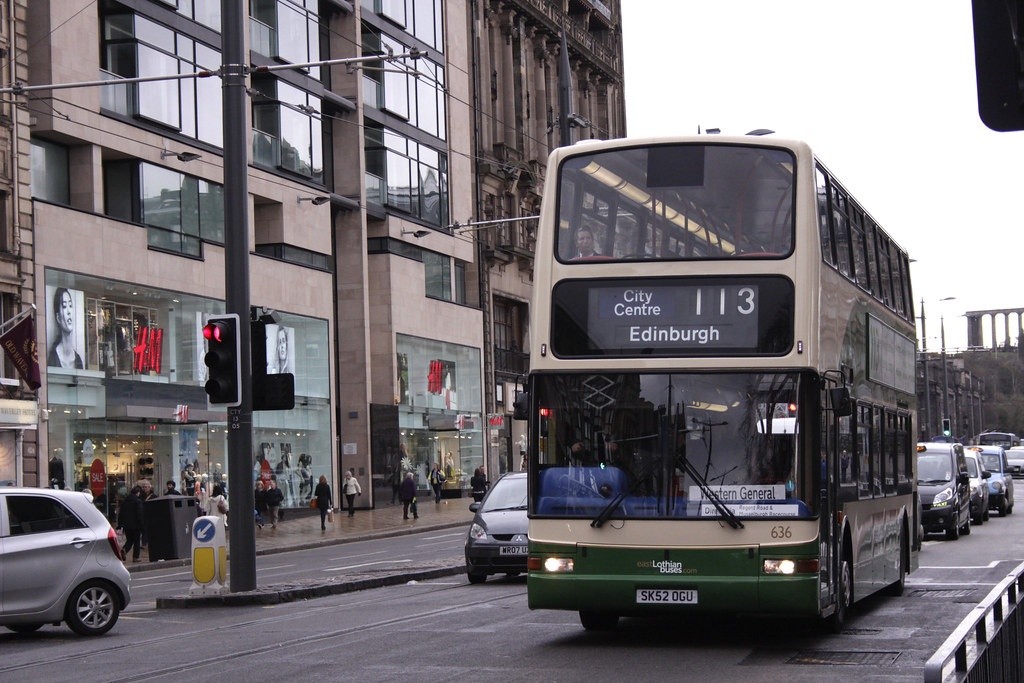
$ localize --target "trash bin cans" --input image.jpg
[144,493,199,563]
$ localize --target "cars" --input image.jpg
[917,441,970,539]
[0,486,131,636]
[962,450,989,524]
[964,444,1014,517]
[466,471,529,583]
[1004,449,1024,478]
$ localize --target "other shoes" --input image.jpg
[141,546,146,549]
[133,558,142,562]
[120,548,126,561]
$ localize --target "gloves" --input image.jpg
[358,493,362,496]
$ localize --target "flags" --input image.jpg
[0,313,42,391]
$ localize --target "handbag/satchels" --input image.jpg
[343,478,347,494]
[311,496,317,509]
[328,505,334,522]
[439,469,445,481]
[217,494,227,514]
[296,469,302,476]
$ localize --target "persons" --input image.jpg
[117,479,181,563]
[471,465,486,502]
[47,287,83,368]
[255,480,267,528]
[344,471,362,517]
[390,470,403,504]
[267,480,283,529]
[254,450,313,508]
[315,475,332,531]
[400,472,420,520]
[272,326,290,373]
[206,485,229,527]
[49,448,66,490]
[444,451,455,478]
[571,374,669,489]
[445,365,451,410]
[428,462,445,504]
[181,461,227,499]
[515,435,527,472]
[573,226,601,257]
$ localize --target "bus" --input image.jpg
[527,135,916,634]
[980,433,1020,450]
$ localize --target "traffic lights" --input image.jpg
[943,419,952,438]
[203,312,242,409]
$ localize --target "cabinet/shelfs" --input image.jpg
[85,296,158,376]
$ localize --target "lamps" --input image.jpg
[401,429,408,436]
[454,432,459,439]
[199,425,306,437]
[48,405,83,414]
[468,433,474,439]
[582,157,737,254]
[434,433,439,439]
[401,226,432,239]
[159,147,201,164]
[460,432,467,439]
[298,193,333,207]
[409,429,416,436]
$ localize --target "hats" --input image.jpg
[167,480,176,487]
[184,464,194,468]
